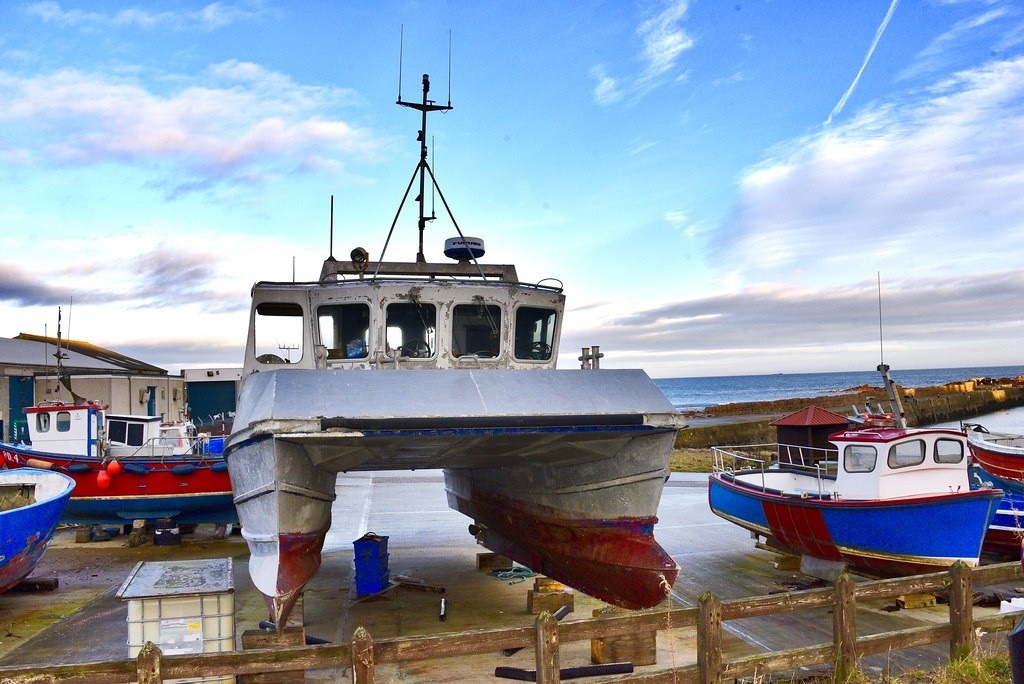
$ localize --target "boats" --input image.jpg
[0,304,239,525]
[222,23,686,631]
[706,427,1005,577]
[965,426,1024,559]
[0,468,78,597]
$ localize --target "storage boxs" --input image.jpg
[346,339,367,358]
[203,438,224,456]
[353,536,391,598]
